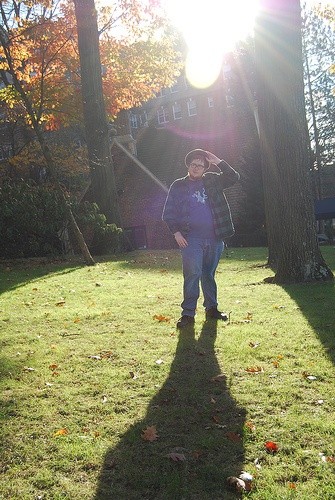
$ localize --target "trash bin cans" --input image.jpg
[124,225,148,251]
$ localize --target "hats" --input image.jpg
[185,149,210,170]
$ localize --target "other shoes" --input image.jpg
[177,316,194,328]
[206,308,228,320]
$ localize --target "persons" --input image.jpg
[162,148,240,326]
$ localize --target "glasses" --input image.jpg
[189,163,206,170]
